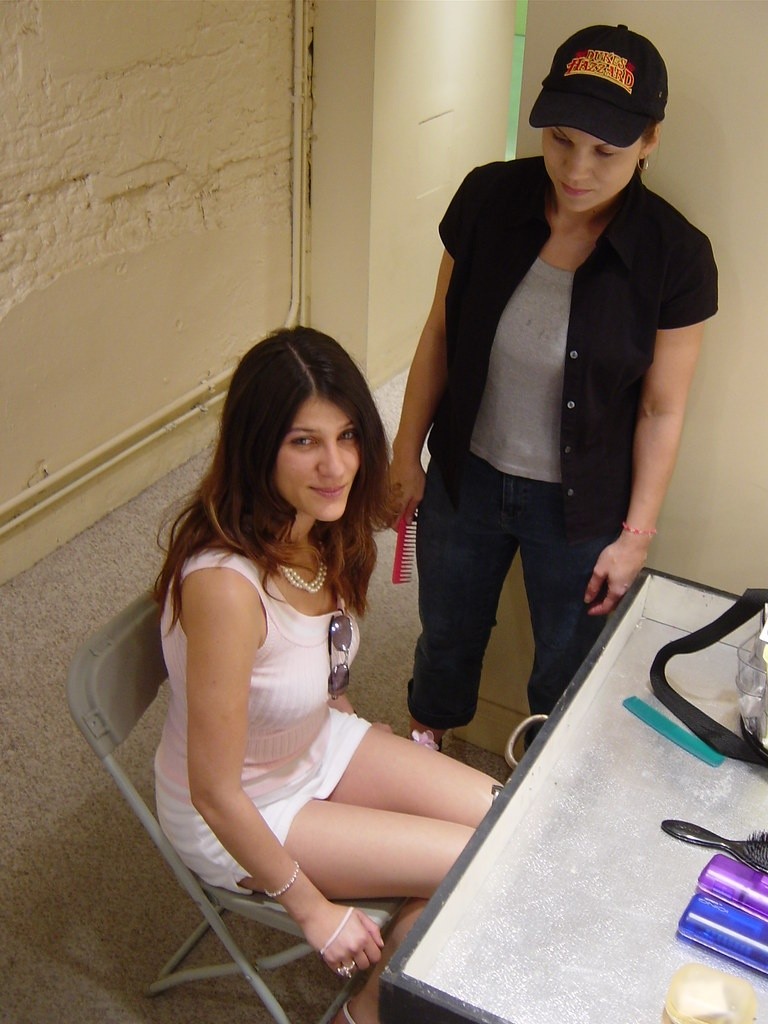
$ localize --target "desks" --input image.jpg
[372,565,768,1024]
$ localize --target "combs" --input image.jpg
[660,817,768,877]
[390,494,422,589]
[622,695,728,770]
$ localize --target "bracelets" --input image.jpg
[264,860,303,899]
[622,522,660,539]
[319,907,356,955]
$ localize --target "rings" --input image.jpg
[338,961,356,977]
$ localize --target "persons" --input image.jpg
[150,325,519,1024]
[379,24,720,754]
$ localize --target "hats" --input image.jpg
[529,25,668,148]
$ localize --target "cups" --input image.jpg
[677,854,768,974]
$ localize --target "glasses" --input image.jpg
[328,609,352,700]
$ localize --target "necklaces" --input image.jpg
[271,540,329,595]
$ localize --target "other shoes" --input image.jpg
[343,997,356,1024]
[406,716,447,753]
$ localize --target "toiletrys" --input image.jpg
[675,891,768,979]
[695,852,768,919]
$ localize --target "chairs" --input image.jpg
[65,578,378,1024]
